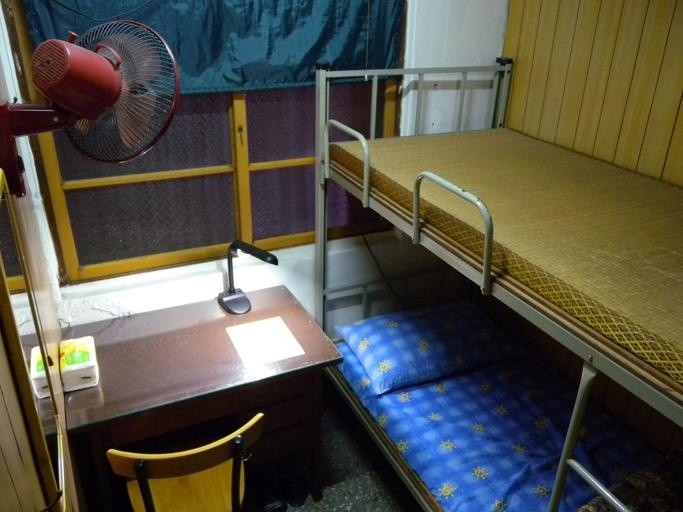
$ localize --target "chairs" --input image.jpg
[105,410,265,511]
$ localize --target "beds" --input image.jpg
[307,57,682,512]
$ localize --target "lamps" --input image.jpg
[217,239,278,315]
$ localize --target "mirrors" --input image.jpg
[1,171,63,508]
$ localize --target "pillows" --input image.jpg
[335,306,520,398]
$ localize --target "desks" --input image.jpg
[61,285,345,503]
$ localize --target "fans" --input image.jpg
[2,21,180,200]
